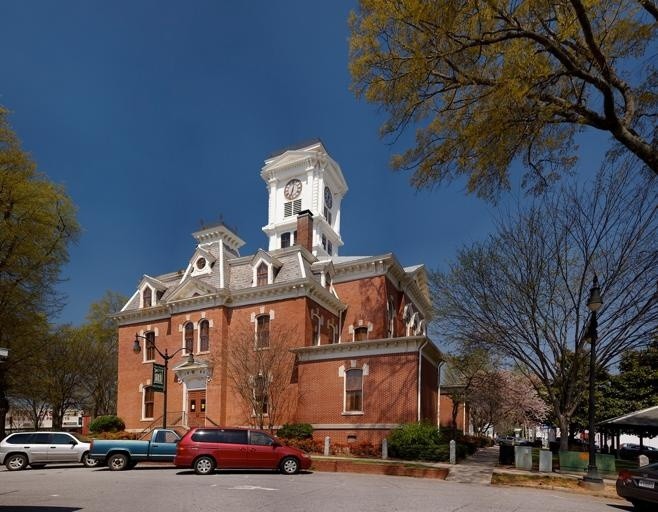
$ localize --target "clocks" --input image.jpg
[283,179,303,200]
[324,186,333,209]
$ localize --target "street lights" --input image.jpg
[0,347,12,441]
[134,332,195,428]
[579,273,607,490]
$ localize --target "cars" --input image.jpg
[556,435,658,512]
[496,434,548,447]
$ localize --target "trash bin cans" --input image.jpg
[516,446,532,471]
[539,449,553,472]
